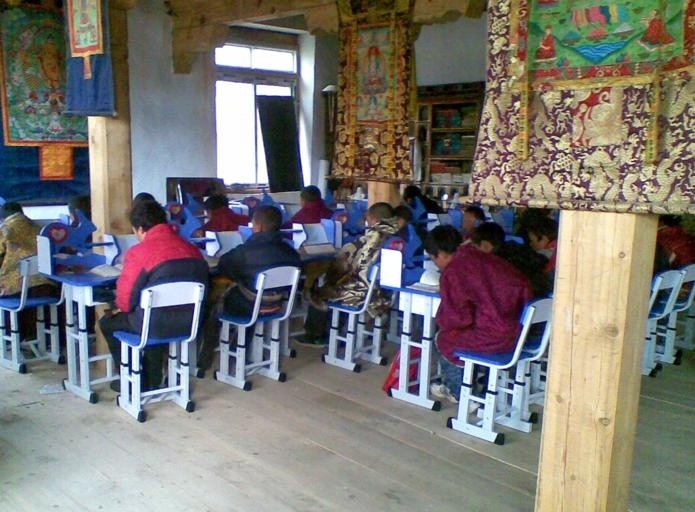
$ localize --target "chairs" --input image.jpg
[447,296,554,446]
[215,265,301,392]
[112,283,205,422]
[640,269,683,378]
[323,259,386,373]
[656,263,693,363]
[2,250,65,373]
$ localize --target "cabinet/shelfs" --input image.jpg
[411,94,481,193]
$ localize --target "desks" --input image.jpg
[382,244,463,411]
[37,229,121,405]
[275,219,342,358]
[33,192,510,233]
[183,225,253,380]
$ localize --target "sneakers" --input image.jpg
[110,379,144,393]
[294,335,325,348]
[469,401,480,414]
[431,383,460,403]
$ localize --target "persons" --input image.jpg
[293,201,401,348]
[657,214,695,300]
[393,204,427,287]
[98,193,210,395]
[527,215,558,286]
[461,204,488,244]
[218,205,303,319]
[403,184,444,214]
[0,200,59,298]
[192,193,251,237]
[281,184,332,241]
[423,223,534,404]
[472,221,554,298]
[59,195,92,256]
